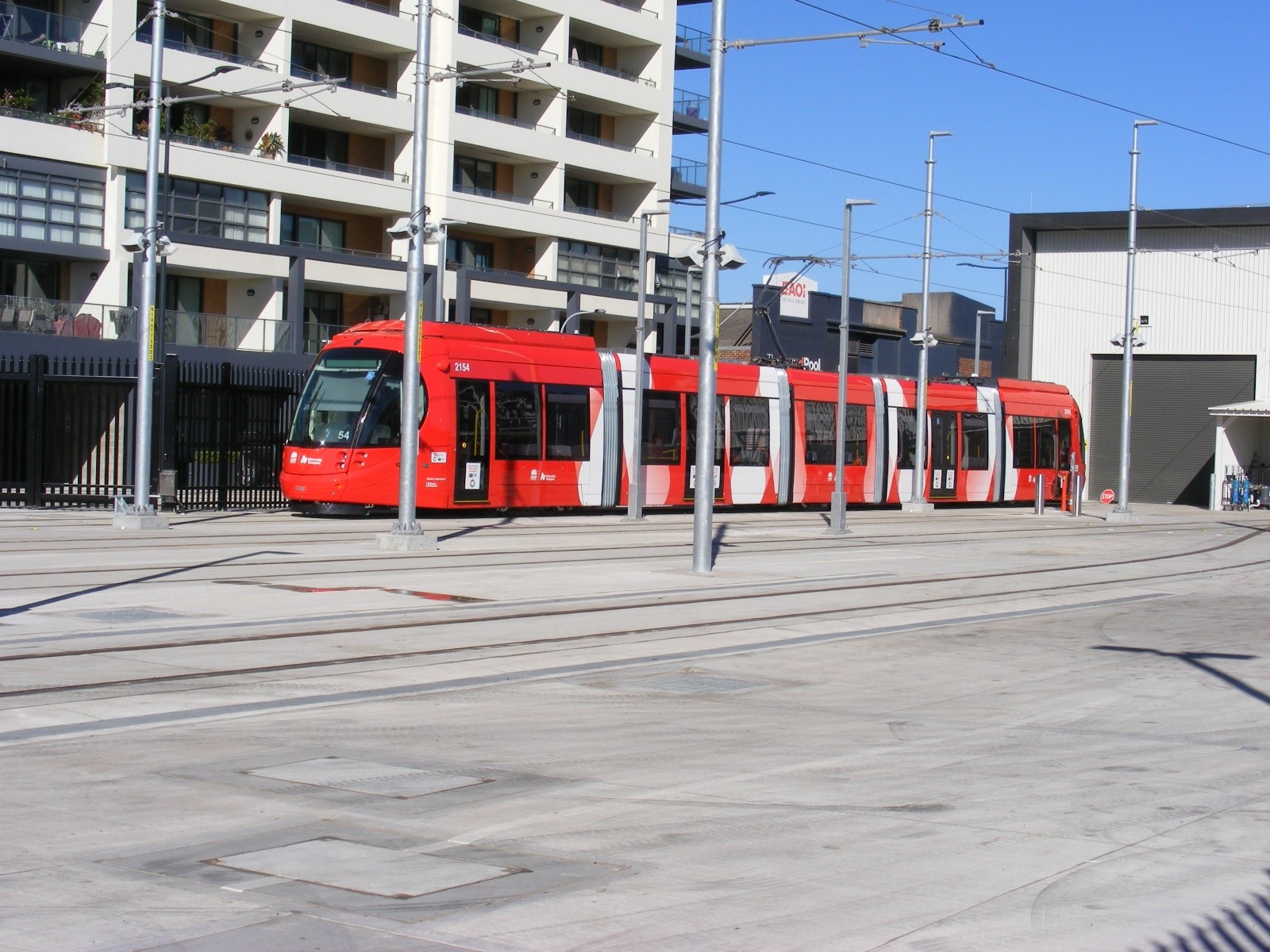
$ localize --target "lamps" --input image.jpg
[525,245,533,252]
[251,117,259,124]
[531,171,538,179]
[255,31,263,38]
[90,272,97,280]
[536,25,543,33]
[247,289,255,296]
[526,318,535,324]
[533,99,541,105]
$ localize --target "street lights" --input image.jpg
[902,131,953,512]
[436,217,467,321]
[1112,119,1158,512]
[101,65,242,364]
[823,199,876,535]
[956,262,1009,322]
[974,310,996,374]
[685,266,702,357]
[628,209,671,520]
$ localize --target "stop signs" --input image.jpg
[1100,489,1114,504]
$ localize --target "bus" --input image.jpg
[278,316,1084,519]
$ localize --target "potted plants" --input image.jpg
[188,452,242,487]
[257,132,284,159]
